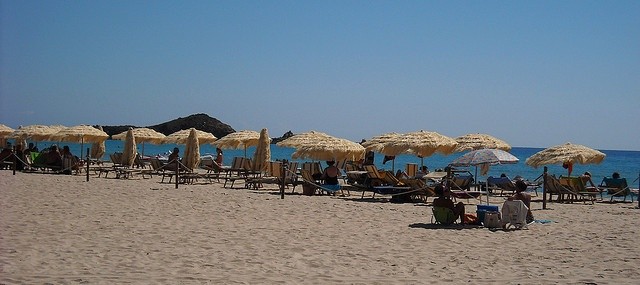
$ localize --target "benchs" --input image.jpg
[124,169,154,180]
[98,166,116,178]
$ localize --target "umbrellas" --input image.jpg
[524,143,606,185]
[362,130,458,169]
[111,127,165,158]
[21,123,70,150]
[274,130,332,150]
[181,129,200,173]
[451,149,519,206]
[210,130,272,158]
[162,127,218,144]
[289,138,365,161]
[453,133,511,185]
[53,126,110,157]
[252,128,270,178]
[123,127,137,168]
[1,124,13,139]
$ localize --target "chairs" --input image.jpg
[605,177,639,202]
[72,155,99,174]
[344,171,371,196]
[299,169,352,196]
[224,168,248,189]
[148,158,166,171]
[502,200,529,231]
[362,165,384,198]
[378,170,405,187]
[279,161,299,193]
[572,176,607,204]
[491,177,542,196]
[243,158,263,188]
[546,173,577,203]
[159,163,198,183]
[303,162,324,194]
[25,152,62,172]
[399,177,453,203]
[269,162,289,193]
[432,207,457,225]
[443,171,475,203]
[207,157,244,184]
[442,179,487,204]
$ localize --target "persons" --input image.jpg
[611,172,620,179]
[396,169,436,196]
[211,146,223,172]
[512,180,534,223]
[24,143,38,155]
[16,144,24,172]
[433,183,464,225]
[579,171,597,200]
[62,145,70,167]
[0,142,12,170]
[319,161,342,196]
[134,152,143,167]
[33,148,49,171]
[501,174,542,186]
[45,144,62,167]
[160,147,179,183]
[415,166,428,178]
